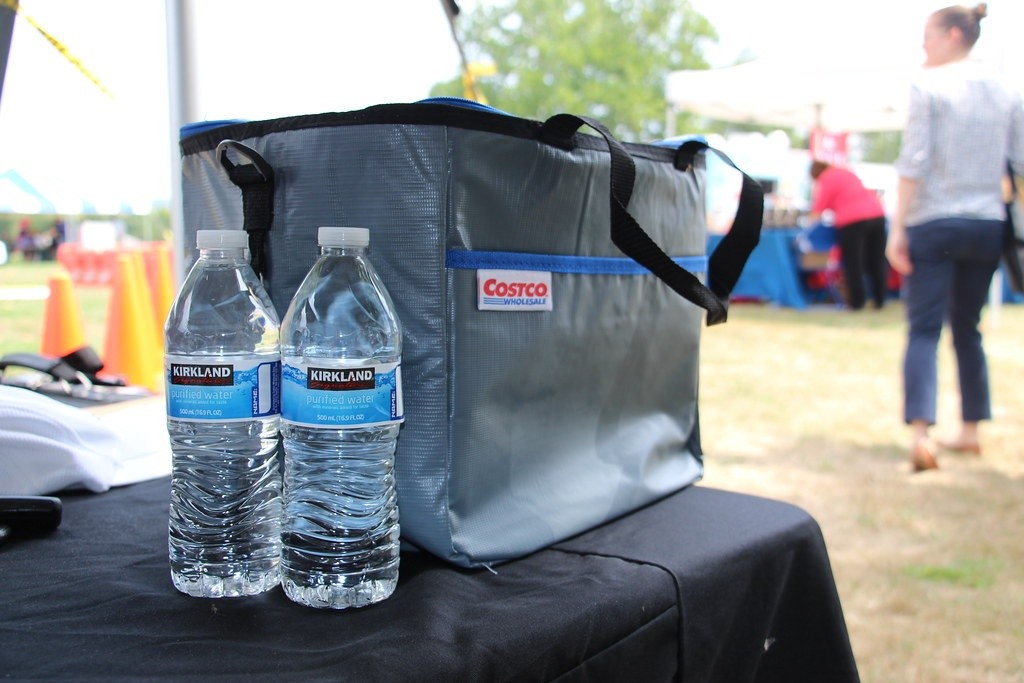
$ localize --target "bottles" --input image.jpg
[162,228,281,599]
[281,224,406,613]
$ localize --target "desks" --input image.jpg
[705,223,847,311]
[0,454,860,683]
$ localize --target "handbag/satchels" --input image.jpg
[0,346,173,497]
[176,98,765,571]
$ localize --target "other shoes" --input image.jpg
[937,440,981,454]
[912,444,938,471]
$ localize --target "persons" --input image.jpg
[17,215,65,263]
[885,2,1024,472]
[809,161,887,311]
